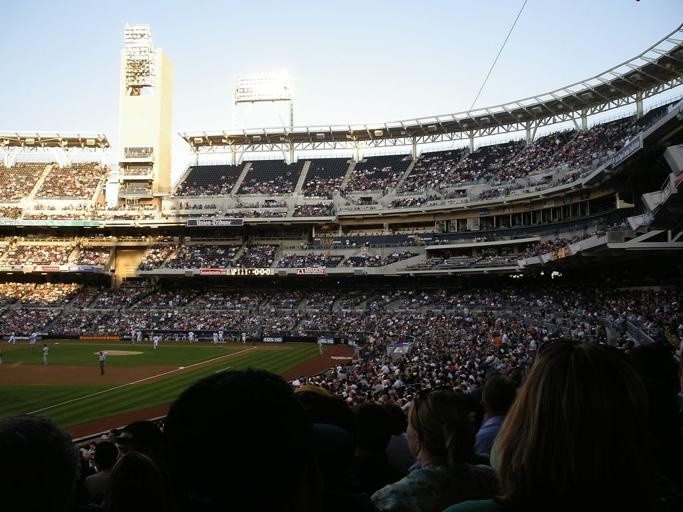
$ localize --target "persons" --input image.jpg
[98,352,106,376]
[1,368,682,511]
[389,120,644,207]
[42,344,50,366]
[0,279,259,351]
[1,161,154,221]
[278,219,630,268]
[255,286,680,412]
[1,227,276,268]
[160,166,406,218]
[317,336,325,354]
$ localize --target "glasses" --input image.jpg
[537,334,608,353]
[414,385,453,427]
